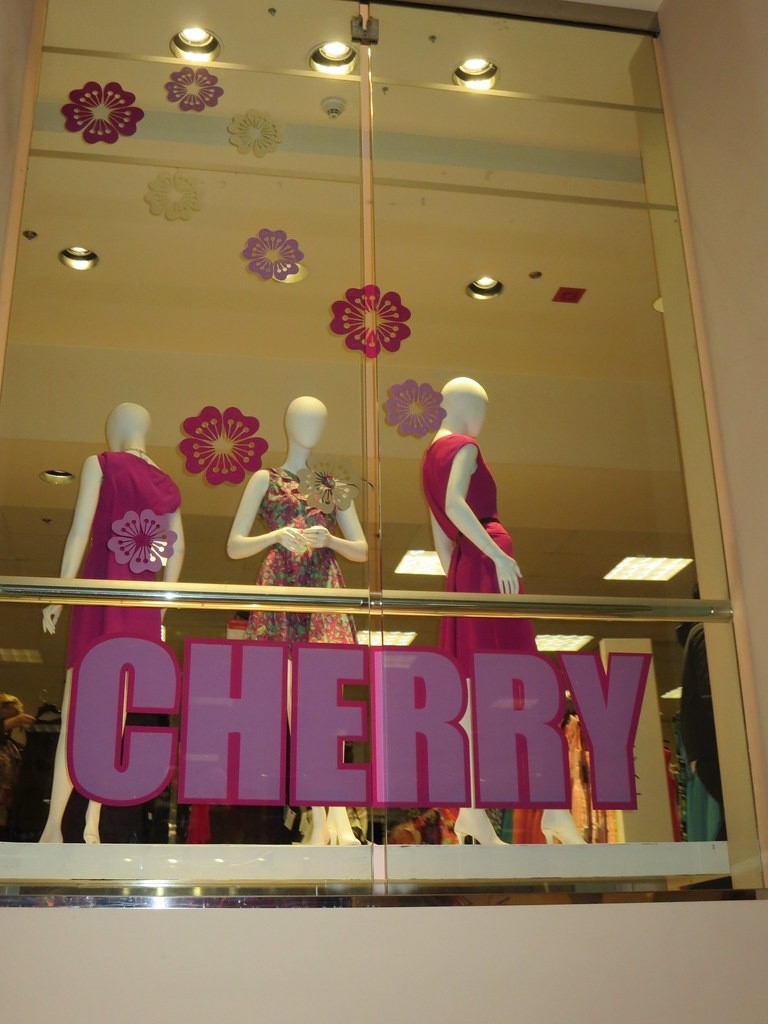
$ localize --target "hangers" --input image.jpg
[32,690,61,725]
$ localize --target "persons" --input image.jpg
[38,402,185,844]
[1,695,37,842]
[226,395,368,846]
[423,377,589,846]
